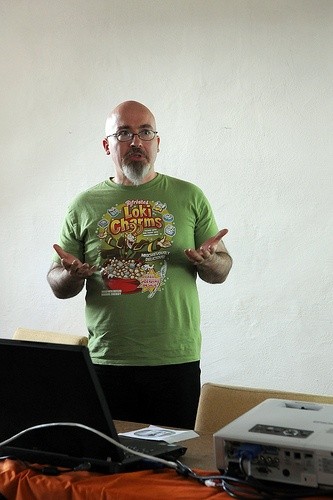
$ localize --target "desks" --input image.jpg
[0,419,252,500]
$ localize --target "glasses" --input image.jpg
[105,129,158,142]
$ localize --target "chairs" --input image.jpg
[13,326,89,346]
[194,381,333,433]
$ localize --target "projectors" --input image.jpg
[213,398,333,489]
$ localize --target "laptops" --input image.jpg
[0,338,187,474]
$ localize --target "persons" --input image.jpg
[46,99,234,432]
